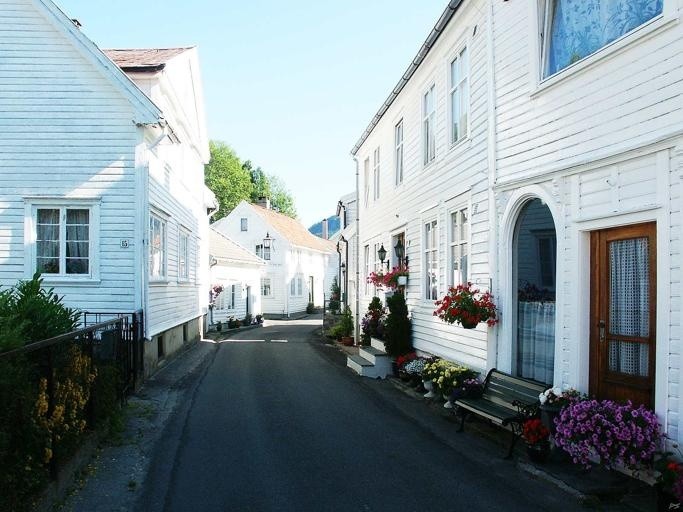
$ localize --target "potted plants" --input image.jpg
[227,316,235,329]
[330,320,343,342]
[216,321,222,331]
[382,290,414,378]
[236,319,240,328]
[243,313,252,326]
[340,306,354,345]
[330,277,340,300]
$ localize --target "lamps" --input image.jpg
[394,239,408,267]
[378,246,389,270]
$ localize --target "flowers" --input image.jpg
[366,269,384,290]
[433,282,499,327]
[396,352,481,398]
[522,418,550,446]
[551,397,664,471]
[384,266,409,289]
[656,452,683,501]
[539,383,590,408]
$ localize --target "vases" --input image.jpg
[443,393,458,409]
[658,487,683,512]
[527,440,551,464]
[379,276,385,284]
[540,405,567,436]
[553,436,662,488]
[397,276,408,286]
[461,315,479,328]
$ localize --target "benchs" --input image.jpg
[450,368,553,460]
